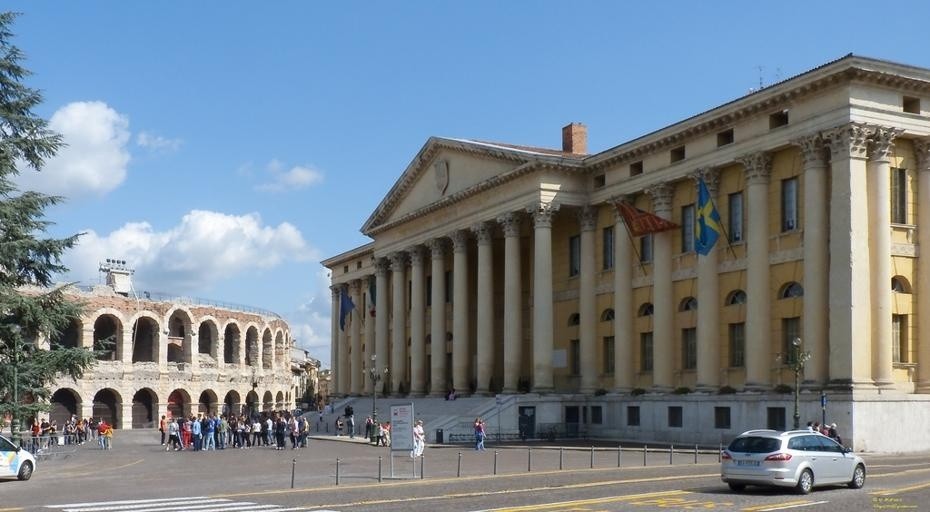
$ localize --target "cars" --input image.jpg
[721,429,866,494]
[0,435,36,480]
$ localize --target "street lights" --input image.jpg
[0,324,35,448]
[363,354,388,442]
[776,337,812,429]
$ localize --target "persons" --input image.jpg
[806,421,842,445]
[410,419,426,459]
[1,410,13,437]
[311,398,335,413]
[159,409,310,452]
[316,412,324,422]
[472,418,488,451]
[335,414,391,447]
[29,413,114,458]
[444,388,457,400]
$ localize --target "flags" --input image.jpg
[615,195,682,238]
[694,178,722,256]
[366,279,376,318]
[339,289,356,332]
